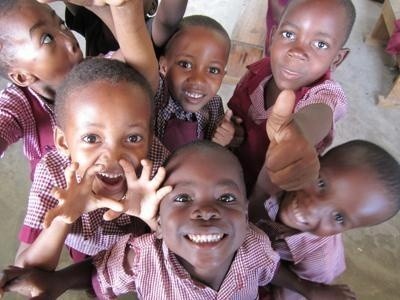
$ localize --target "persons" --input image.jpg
[13,58,174,300]
[252,140,400,300]
[37,1,245,181]
[0,0,127,183]
[65,1,188,60]
[226,0,356,210]
[0,141,360,300]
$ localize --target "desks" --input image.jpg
[364,0,400,109]
